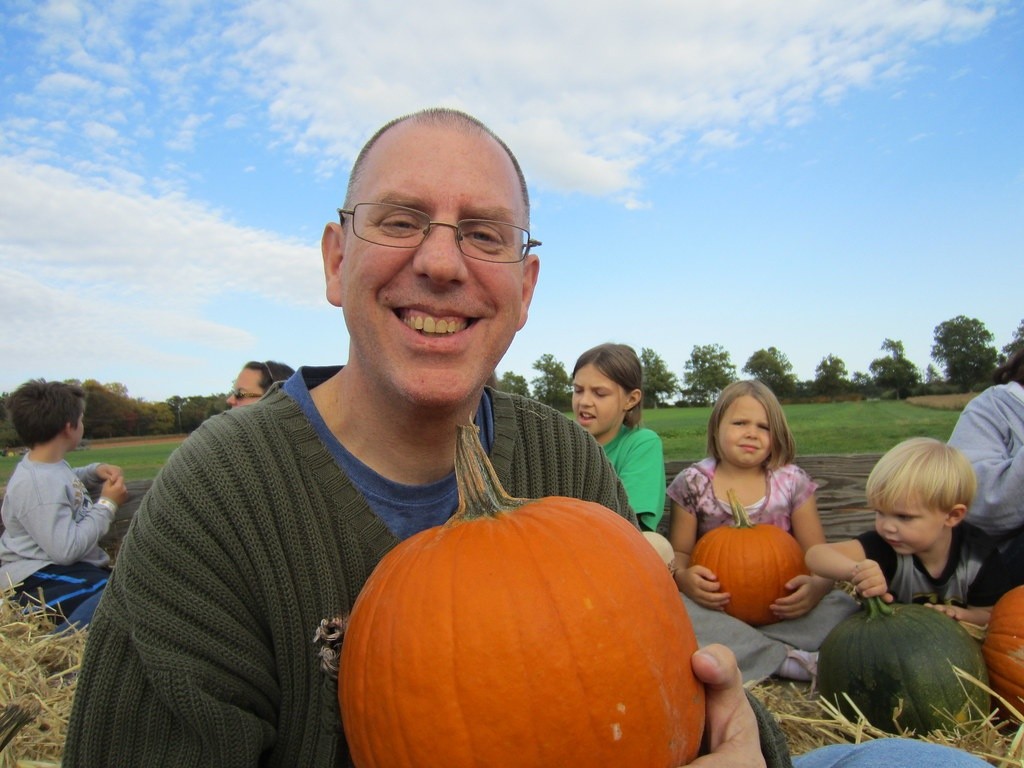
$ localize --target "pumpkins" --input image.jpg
[819,590,990,744]
[955,585,1024,721]
[691,486,803,622]
[336,422,710,768]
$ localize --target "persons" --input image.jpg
[664,379,862,691]
[59,106,998,768]
[941,334,1024,603]
[225,359,299,410]
[571,342,666,533]
[804,435,1012,629]
[0,380,128,636]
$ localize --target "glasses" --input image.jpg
[231,390,263,400]
[337,201,543,264]
